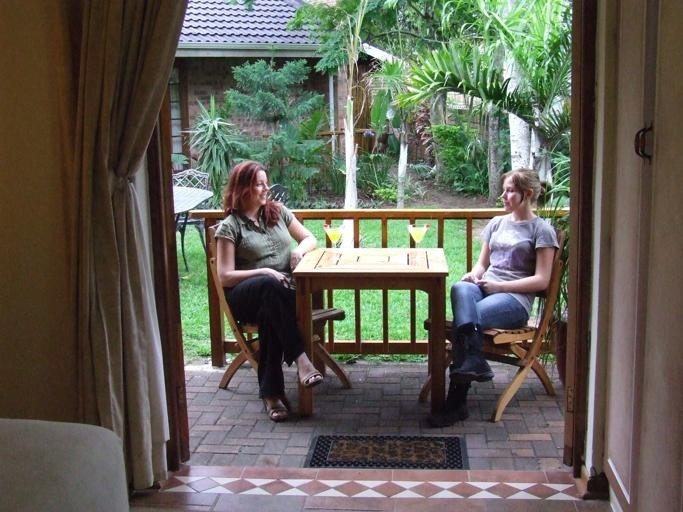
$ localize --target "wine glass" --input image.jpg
[406,224,429,261]
[324,225,344,259]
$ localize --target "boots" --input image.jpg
[450,321,494,385]
[426,364,469,427]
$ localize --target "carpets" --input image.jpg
[303,435,469,470]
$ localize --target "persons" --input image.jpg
[212,160,325,422]
[423,167,559,428]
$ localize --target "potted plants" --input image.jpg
[548,131,570,387]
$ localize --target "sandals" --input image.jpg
[299,367,324,388]
[264,395,289,420]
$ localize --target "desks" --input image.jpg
[291,245,449,417]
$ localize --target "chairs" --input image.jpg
[173,170,213,273]
[418,232,569,422]
[203,225,351,392]
